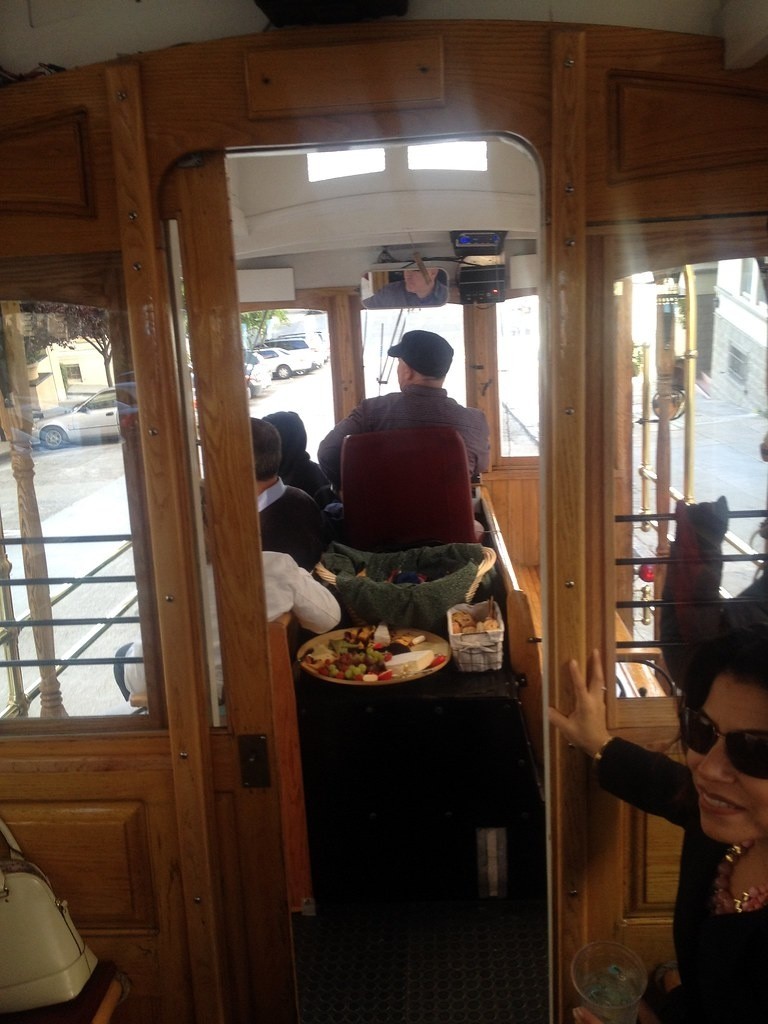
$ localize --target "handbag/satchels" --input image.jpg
[1,816,99,1014]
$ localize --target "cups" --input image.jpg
[568,943,649,1024]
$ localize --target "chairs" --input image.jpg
[341,428,483,552]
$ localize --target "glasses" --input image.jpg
[673,699,768,780]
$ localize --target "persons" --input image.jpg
[362,269,447,307]
[113,479,342,703]
[544,625,768,1024]
[249,416,324,572]
[318,327,489,504]
[260,410,335,511]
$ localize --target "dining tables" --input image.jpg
[298,630,546,900]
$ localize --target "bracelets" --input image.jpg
[592,736,614,762]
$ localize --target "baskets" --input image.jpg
[313,542,497,631]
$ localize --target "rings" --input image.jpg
[601,687,607,691]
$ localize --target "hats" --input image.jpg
[386,329,457,380]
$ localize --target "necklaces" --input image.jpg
[710,840,767,915]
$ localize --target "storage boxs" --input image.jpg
[315,544,497,632]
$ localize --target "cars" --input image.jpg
[26,325,336,457]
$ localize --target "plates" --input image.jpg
[295,623,452,687]
